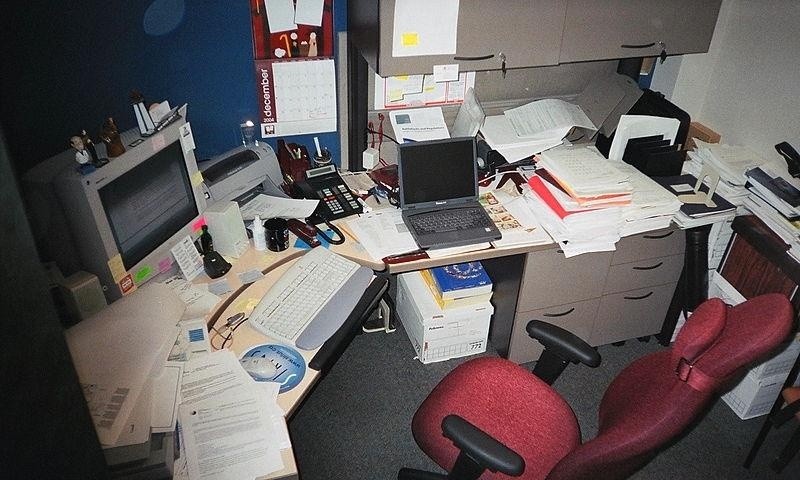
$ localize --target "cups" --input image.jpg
[239,122,256,149]
[312,148,333,170]
[262,217,291,252]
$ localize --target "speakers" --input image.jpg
[58,270,108,326]
[203,200,250,259]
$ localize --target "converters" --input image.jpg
[362,148,380,170]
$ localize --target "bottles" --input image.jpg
[200,224,213,255]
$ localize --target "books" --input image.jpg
[478,178,548,250]
[417,260,494,311]
[650,173,737,230]
[742,167,800,265]
[516,144,634,224]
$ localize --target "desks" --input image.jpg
[169,184,558,479]
[717,214,799,305]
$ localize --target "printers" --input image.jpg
[199,140,294,239]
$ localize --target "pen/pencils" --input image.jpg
[313,137,323,157]
[337,223,360,244]
[285,143,301,160]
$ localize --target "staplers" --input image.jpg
[287,219,321,248]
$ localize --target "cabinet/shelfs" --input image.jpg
[487,227,690,372]
[341,0,727,77]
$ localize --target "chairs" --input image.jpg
[394,288,795,478]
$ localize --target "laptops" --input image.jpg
[396,137,502,251]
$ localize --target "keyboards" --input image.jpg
[246,245,374,351]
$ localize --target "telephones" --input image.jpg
[294,163,363,225]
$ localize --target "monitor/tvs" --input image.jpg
[53,119,207,301]
